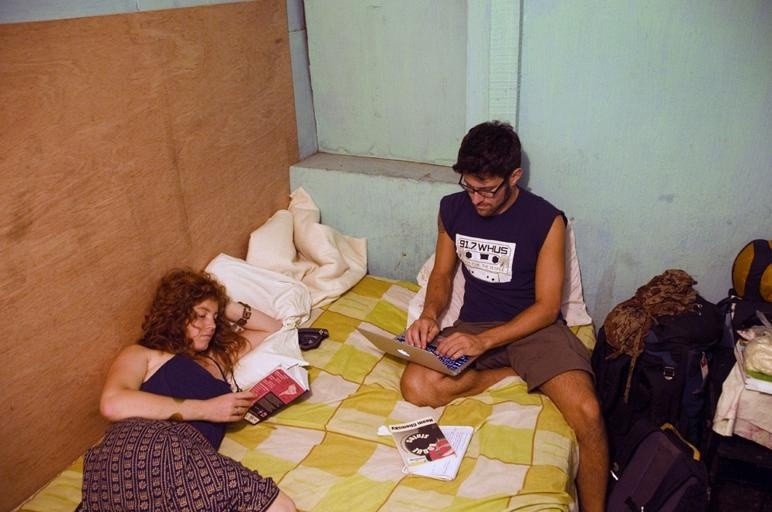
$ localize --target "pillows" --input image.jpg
[205,253,313,325]
[407,221,592,331]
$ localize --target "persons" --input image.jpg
[75,264,296,512]
[401,121,610,511]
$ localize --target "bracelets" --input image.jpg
[234,301,252,327]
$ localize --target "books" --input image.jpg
[388,415,457,470]
[733,340,772,396]
[242,365,310,426]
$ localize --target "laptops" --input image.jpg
[353,324,480,377]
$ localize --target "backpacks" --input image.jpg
[605,420,712,512]
[590,284,743,487]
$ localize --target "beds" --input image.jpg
[13,272,598,512]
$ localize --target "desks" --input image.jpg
[712,361,772,448]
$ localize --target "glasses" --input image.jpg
[458,173,505,198]
